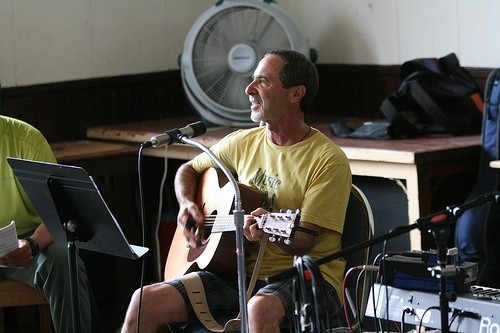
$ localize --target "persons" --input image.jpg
[0,115,92,333]
[121,48,352,333]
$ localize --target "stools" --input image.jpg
[0,279,54,333]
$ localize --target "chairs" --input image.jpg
[166,183,375,333]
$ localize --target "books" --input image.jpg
[0,221,25,270]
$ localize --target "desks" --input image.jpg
[50,139,141,305]
[86,115,481,252]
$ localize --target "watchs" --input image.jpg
[24,237,40,259]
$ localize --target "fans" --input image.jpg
[177,0,318,129]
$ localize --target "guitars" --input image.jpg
[164,166,303,281]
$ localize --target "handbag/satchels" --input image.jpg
[380,53,483,141]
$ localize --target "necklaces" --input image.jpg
[299,125,311,142]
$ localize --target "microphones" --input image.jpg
[140,120,207,149]
